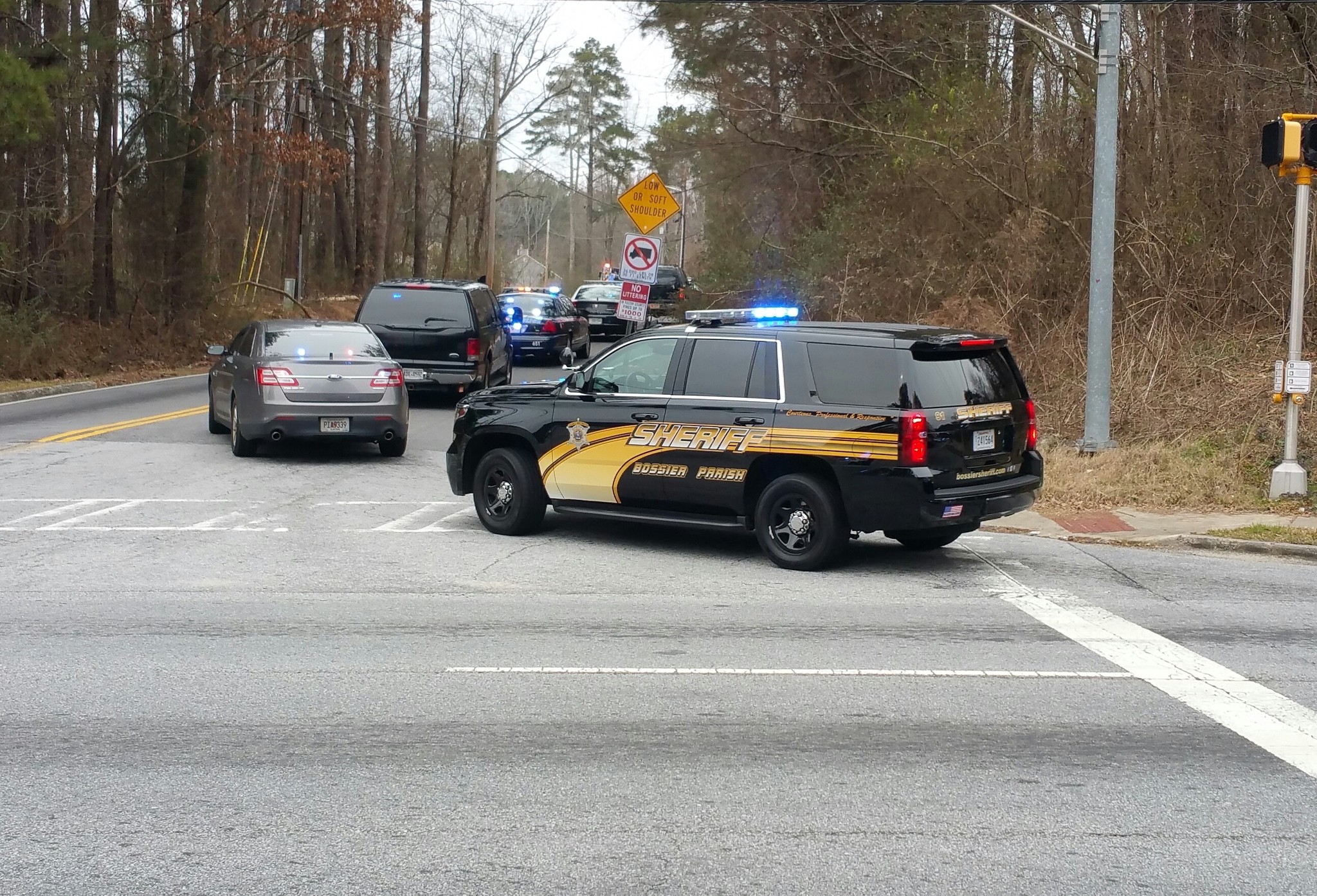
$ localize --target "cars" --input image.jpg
[688,277,704,301]
[207,318,409,458]
[495,286,591,365]
[570,279,653,341]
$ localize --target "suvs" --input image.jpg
[597,262,693,326]
[356,276,518,405]
[446,306,1045,573]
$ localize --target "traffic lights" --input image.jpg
[1303,119,1317,169]
[1262,119,1301,168]
